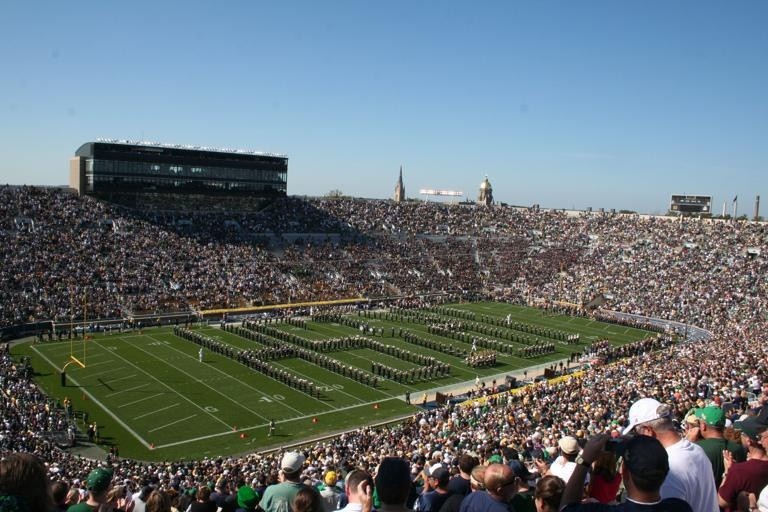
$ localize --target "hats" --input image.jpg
[87,466,113,495]
[325,471,337,485]
[280,452,305,473]
[734,416,768,439]
[378,456,410,481]
[695,407,726,427]
[621,436,669,479]
[238,485,259,508]
[428,467,449,480]
[623,397,670,436]
[560,437,579,455]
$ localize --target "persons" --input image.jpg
[1,183,767,512]
